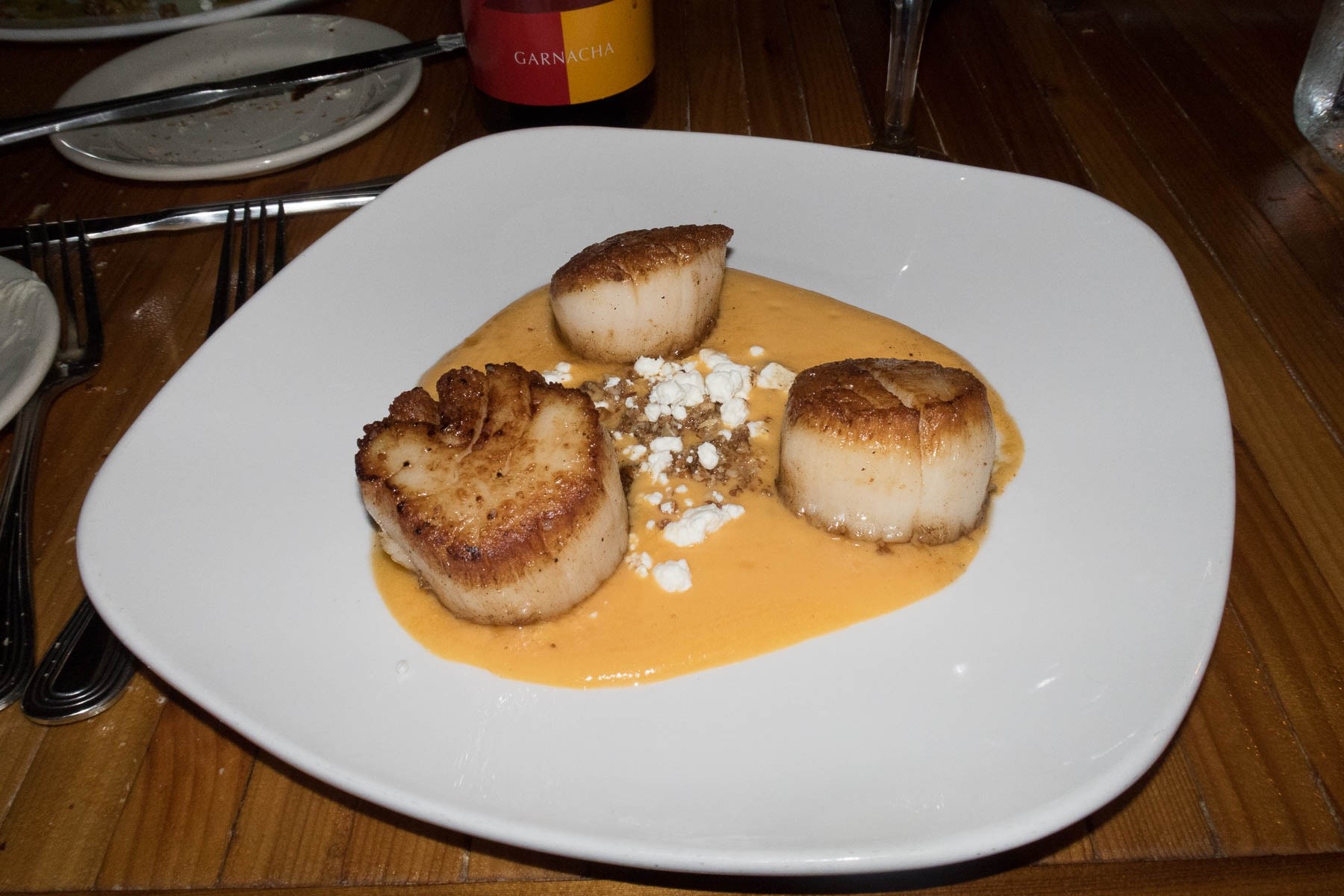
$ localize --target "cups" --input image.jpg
[1292,0,1344,175]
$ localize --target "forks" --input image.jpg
[0,208,104,714]
[22,198,286,726]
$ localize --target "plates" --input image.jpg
[0,0,297,43]
[77,123,1237,879]
[50,15,423,183]
[1,252,62,431]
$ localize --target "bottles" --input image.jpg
[457,0,657,133]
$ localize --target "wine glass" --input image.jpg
[848,1,960,165]
